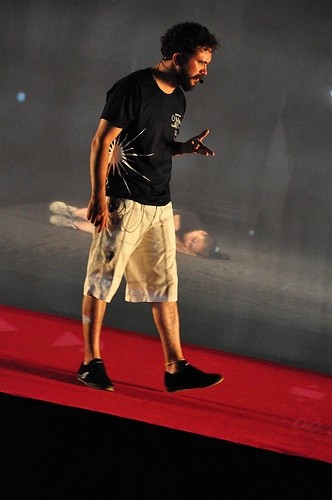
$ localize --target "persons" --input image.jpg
[48,200,231,260]
[75,21,225,393]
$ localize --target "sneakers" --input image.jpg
[49,201,78,218]
[76,359,114,391]
[165,360,224,392]
[50,215,78,230]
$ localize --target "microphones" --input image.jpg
[199,80,203,83]
